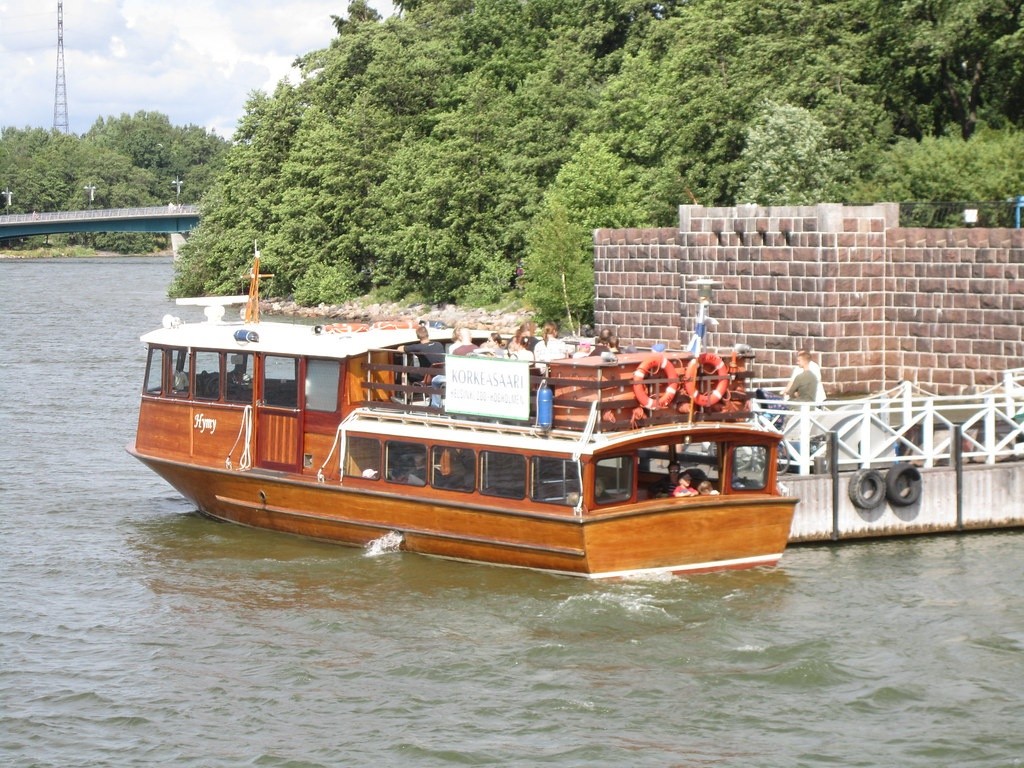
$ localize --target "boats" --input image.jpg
[171,180,184,204]
[124,237,799,578]
[83,186,96,209]
[681,368,1023,543]
[2,191,14,215]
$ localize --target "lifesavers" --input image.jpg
[685,353,727,406]
[634,355,678,410]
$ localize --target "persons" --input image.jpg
[168,362,189,393]
[672,472,700,498]
[385,441,609,509]
[394,316,623,405]
[778,348,829,407]
[213,354,270,405]
[649,462,681,498]
[698,480,719,497]
[779,353,821,461]
